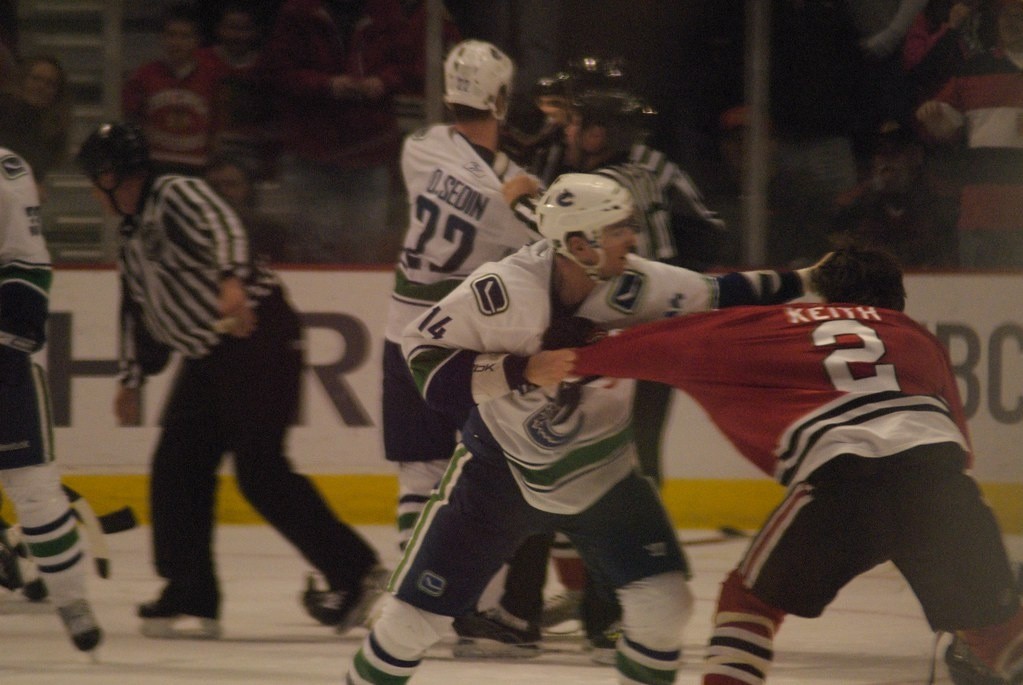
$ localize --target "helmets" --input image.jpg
[535,173,634,241]
[443,39,513,110]
[572,88,657,134]
[75,121,148,166]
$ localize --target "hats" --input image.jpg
[866,121,919,158]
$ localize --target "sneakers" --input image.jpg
[59,599,101,664]
[452,603,543,659]
[305,569,389,629]
[541,590,588,638]
[138,579,221,639]
[590,618,623,666]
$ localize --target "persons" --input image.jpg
[0,1,390,664]
[344,1,1023,684]
